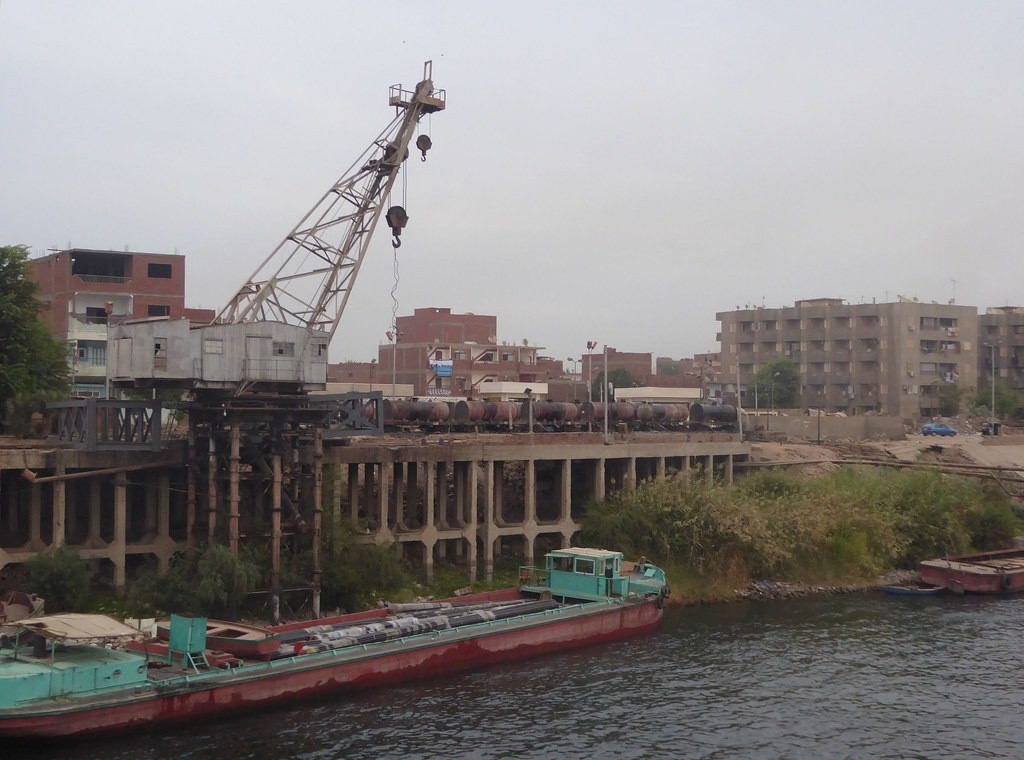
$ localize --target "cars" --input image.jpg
[921,422,957,437]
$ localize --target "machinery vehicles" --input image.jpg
[204,59,449,430]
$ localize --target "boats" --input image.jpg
[1,590,44,647]
[155,617,282,657]
[0,547,671,750]
[920,546,1024,596]
[879,585,948,596]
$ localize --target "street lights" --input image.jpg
[104,301,114,400]
[586,341,598,432]
[386,331,405,400]
[983,340,1003,436]
[567,358,583,400]
